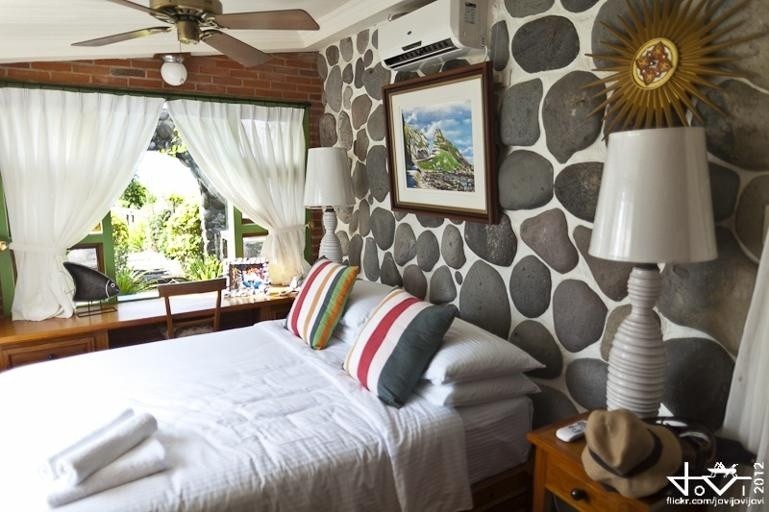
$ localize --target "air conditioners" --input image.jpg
[377,1,489,73]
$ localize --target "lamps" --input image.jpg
[152,52,191,88]
[302,145,356,263]
[584,124,722,419]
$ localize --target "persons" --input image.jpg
[242,264,263,288]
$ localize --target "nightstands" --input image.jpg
[524,407,714,511]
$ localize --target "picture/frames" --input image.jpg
[380,59,501,226]
[222,255,272,298]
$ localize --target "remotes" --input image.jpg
[556,418,589,443]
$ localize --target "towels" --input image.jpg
[0,253,550,511]
[46,434,170,507]
[46,405,159,486]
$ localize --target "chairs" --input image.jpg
[157,278,227,339]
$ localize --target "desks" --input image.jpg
[1,277,297,370]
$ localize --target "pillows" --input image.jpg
[421,316,548,385]
[332,324,363,345]
[341,286,458,407]
[412,373,544,408]
[283,254,362,351]
[339,278,393,328]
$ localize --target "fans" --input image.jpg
[70,0,320,69]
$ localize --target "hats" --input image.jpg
[581,409,683,498]
